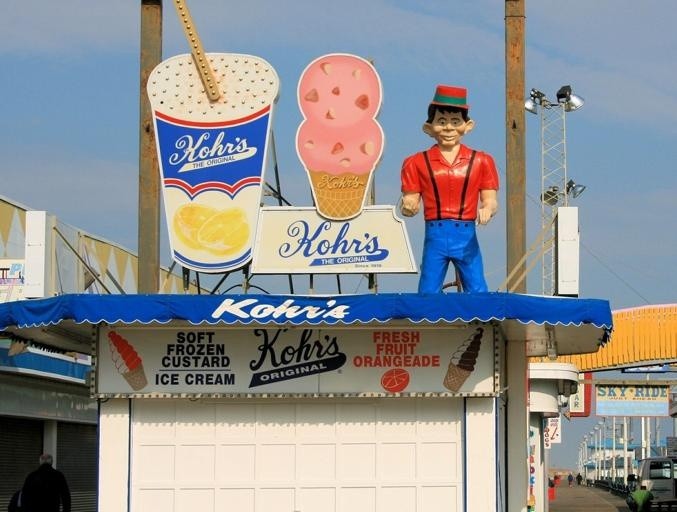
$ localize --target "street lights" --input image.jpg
[578,416,609,487]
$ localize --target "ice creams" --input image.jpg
[107,331,147,392]
[296,52,384,221]
[438,328,484,393]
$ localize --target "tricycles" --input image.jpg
[626,455,676,512]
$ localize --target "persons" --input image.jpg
[401,86,499,292]
[18,454,72,512]
[577,473,582,486]
[554,472,560,486]
[567,473,573,487]
[627,490,654,512]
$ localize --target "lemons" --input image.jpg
[175,206,217,249]
[197,207,249,255]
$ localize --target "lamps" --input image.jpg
[524,83,587,205]
